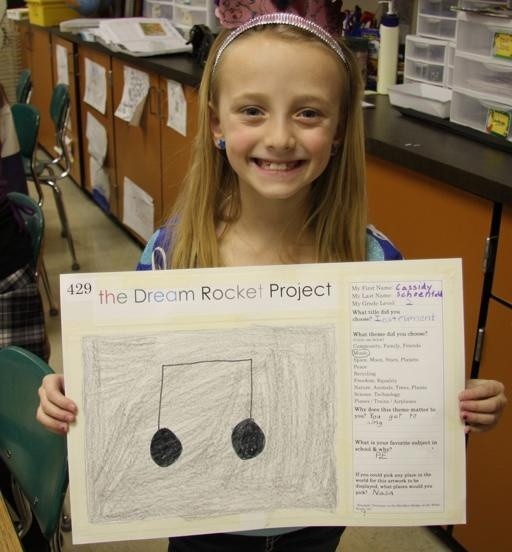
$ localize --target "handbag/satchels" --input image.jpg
[186,24,212,65]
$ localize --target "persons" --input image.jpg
[35,14,507,552]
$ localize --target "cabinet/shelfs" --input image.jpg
[14,22,512,552]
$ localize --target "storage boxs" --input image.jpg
[382,2,511,139]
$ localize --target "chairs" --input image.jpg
[0,68,78,552]
